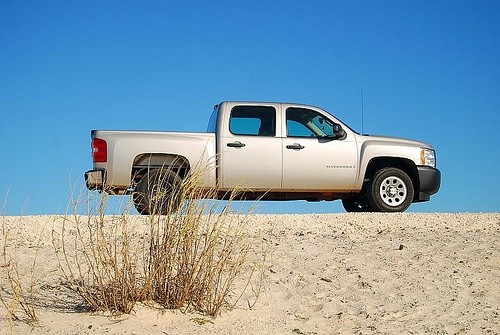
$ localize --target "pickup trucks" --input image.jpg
[84,102,440,215]
